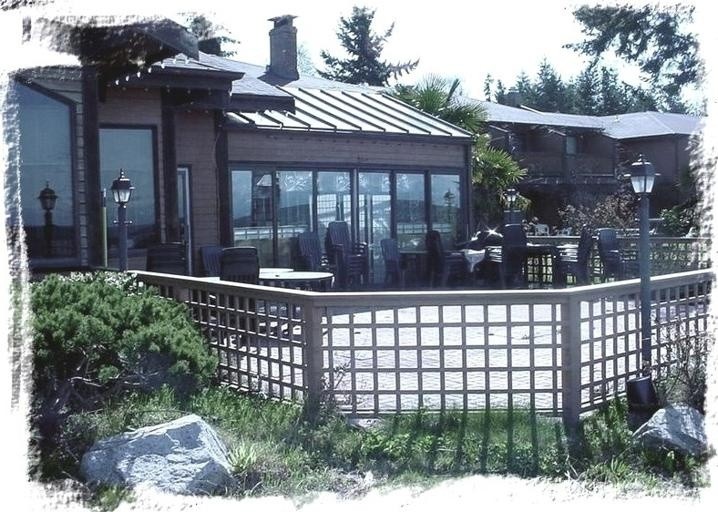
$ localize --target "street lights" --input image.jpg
[624,153,663,401]
[37,179,56,258]
[503,181,517,225]
[444,186,456,232]
[112,168,137,275]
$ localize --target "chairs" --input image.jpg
[137,218,625,324]
